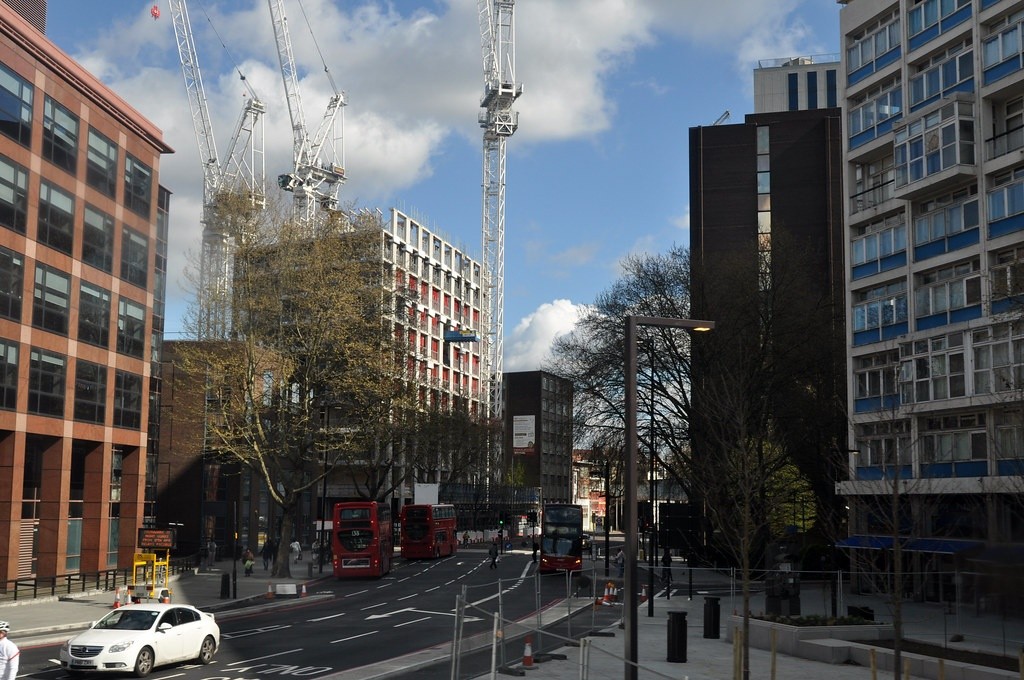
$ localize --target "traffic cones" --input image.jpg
[162,590,170,604]
[595,597,614,608]
[639,584,648,603]
[300,582,309,597]
[602,583,618,603]
[112,589,121,609]
[263,581,275,599]
[124,590,132,605]
[515,637,539,670]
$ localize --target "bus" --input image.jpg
[540,502,584,574]
[330,500,393,579]
[400,503,458,560]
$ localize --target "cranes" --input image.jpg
[261,0,351,237]
[151,0,266,342]
[468,0,527,505]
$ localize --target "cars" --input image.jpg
[60,602,220,678]
[583,534,593,549]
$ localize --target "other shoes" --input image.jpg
[490,567,493,569]
[533,561,537,563]
[495,567,498,569]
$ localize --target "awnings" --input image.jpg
[835,536,985,555]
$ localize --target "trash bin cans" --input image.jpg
[766,568,802,621]
[703,595,721,639]
[666,610,689,663]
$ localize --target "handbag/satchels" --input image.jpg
[298,551,302,560]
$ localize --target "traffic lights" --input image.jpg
[499,513,504,524]
[504,512,511,525]
[526,512,532,523]
[532,512,538,522]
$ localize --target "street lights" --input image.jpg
[622,313,717,680]
[828,448,864,617]
[636,337,655,618]
[319,403,342,575]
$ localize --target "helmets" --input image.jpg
[0,621,10,632]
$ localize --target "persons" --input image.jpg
[311,539,320,561]
[498,529,502,544]
[588,541,592,557]
[207,538,218,566]
[489,543,498,569]
[0,621,20,680]
[326,541,332,564]
[463,532,470,546]
[616,549,624,577]
[661,553,673,581]
[259,537,272,571]
[242,548,254,577]
[532,541,538,563]
[290,539,301,564]
